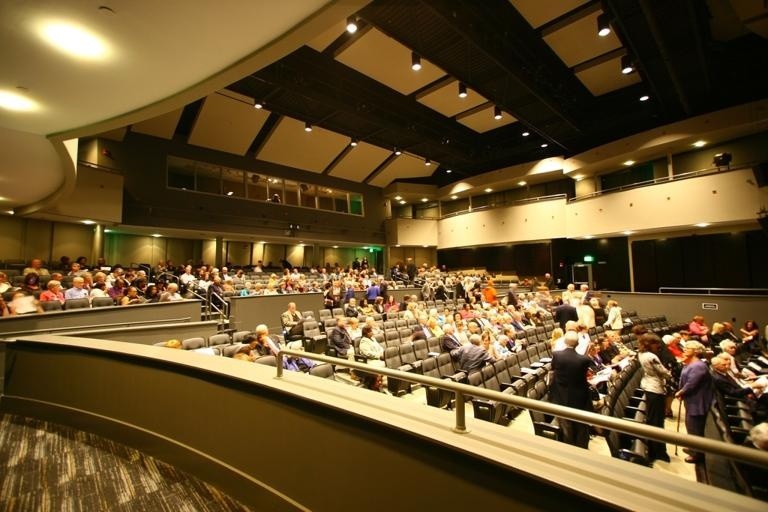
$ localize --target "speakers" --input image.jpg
[752,163,768,187]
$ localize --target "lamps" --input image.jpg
[639,86,648,101]
[494,106,502,120]
[425,158,431,166]
[411,51,422,72]
[621,56,633,75]
[351,137,357,147]
[597,13,610,37]
[347,16,358,34]
[458,82,466,98]
[541,138,548,148]
[394,146,400,156]
[255,97,264,109]
[304,121,312,132]
[522,127,529,137]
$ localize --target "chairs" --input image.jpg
[468,355,527,426]
[300,271,324,288]
[621,311,688,351]
[301,308,350,338]
[153,330,253,357]
[309,362,336,381]
[39,300,62,311]
[375,310,467,408]
[417,299,466,311]
[588,325,611,343]
[245,272,284,289]
[64,297,90,311]
[710,389,766,499]
[516,325,553,403]
[3,269,77,291]
[92,297,114,308]
[601,357,648,463]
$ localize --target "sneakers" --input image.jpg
[685,455,699,462]
[683,448,690,453]
